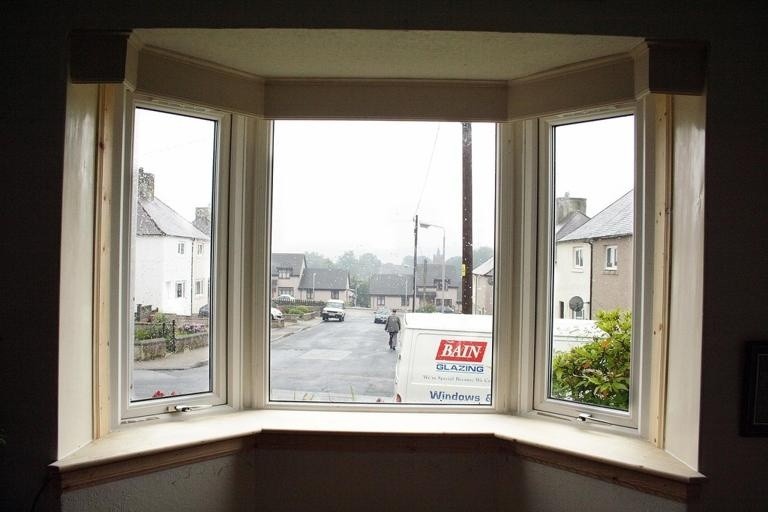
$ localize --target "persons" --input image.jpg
[384,307,401,351]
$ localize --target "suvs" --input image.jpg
[321,299,346,321]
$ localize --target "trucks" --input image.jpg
[390,312,624,407]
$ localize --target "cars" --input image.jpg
[198,304,208,318]
[371,306,391,324]
[272,294,296,304]
[269,307,282,320]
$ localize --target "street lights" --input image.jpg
[419,221,446,315]
[404,264,416,310]
[312,272,315,298]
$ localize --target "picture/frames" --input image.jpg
[735,337,767,444]
